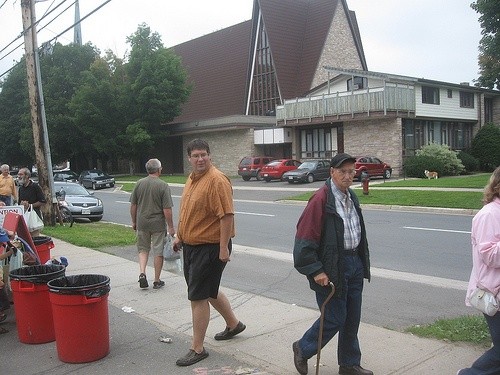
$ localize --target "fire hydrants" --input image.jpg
[361,174,370,195]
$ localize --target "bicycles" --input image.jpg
[53,186,74,227]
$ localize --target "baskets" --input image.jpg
[56,191,66,201]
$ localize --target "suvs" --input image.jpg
[237,155,278,181]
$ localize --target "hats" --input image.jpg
[329,153,357,168]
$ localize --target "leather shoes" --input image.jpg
[293,340,308,375]
[339,365,373,375]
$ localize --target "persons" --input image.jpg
[0,228,13,323]
[172,138,246,367]
[129,159,176,288]
[0,163,17,206]
[18,167,46,237]
[293,153,373,375]
[457,166,500,375]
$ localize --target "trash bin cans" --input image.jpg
[47,273,111,364]
[8,265,66,345]
[33,236,55,264]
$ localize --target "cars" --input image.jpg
[258,158,303,182]
[353,155,392,182]
[53,169,79,183]
[77,169,115,190]
[281,159,331,184]
[53,180,104,224]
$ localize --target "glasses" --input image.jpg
[333,168,356,177]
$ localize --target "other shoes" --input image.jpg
[214,321,246,340]
[153,281,164,289]
[176,347,209,366]
[138,273,148,288]
[0,328,8,334]
[0,314,7,322]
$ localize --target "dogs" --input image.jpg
[424,169,438,179]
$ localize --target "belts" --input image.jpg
[344,248,359,256]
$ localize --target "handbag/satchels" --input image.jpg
[163,234,183,273]
[23,204,44,232]
[163,236,181,261]
[469,287,500,317]
